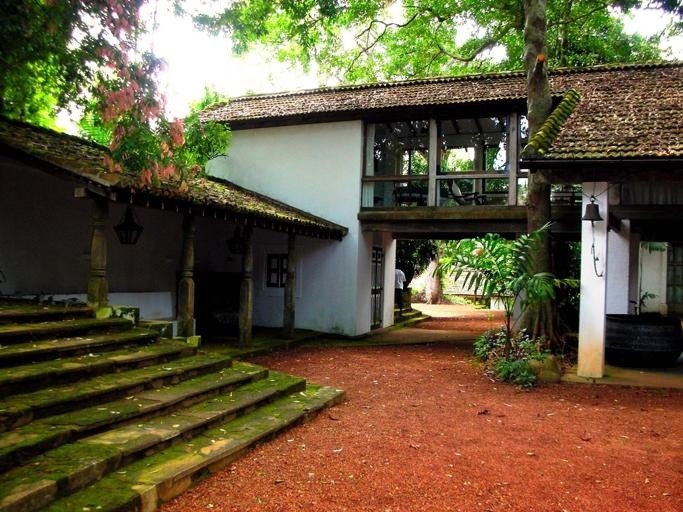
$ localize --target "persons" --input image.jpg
[395,263,407,317]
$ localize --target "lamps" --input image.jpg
[113,203,144,245]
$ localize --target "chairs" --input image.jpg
[446,179,487,206]
